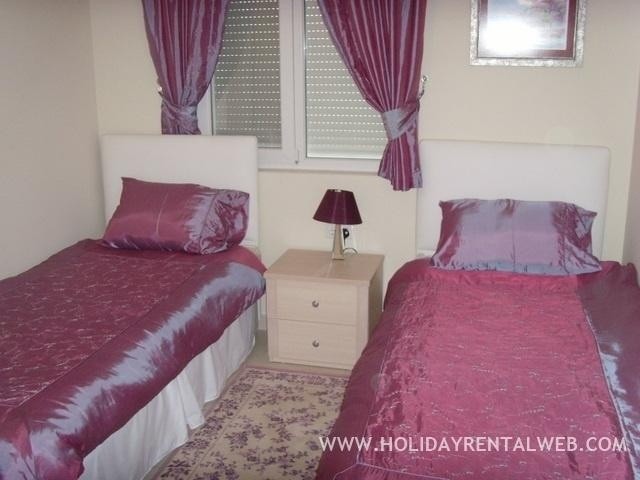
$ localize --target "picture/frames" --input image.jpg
[468,0,587,70]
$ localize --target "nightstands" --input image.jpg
[264,249,386,379]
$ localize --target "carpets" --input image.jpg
[149,361,351,480]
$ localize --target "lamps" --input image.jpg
[312,190,364,260]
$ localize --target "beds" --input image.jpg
[0,132,261,480]
[321,140,639,480]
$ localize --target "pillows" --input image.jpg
[433,200,607,275]
[99,179,252,257]
